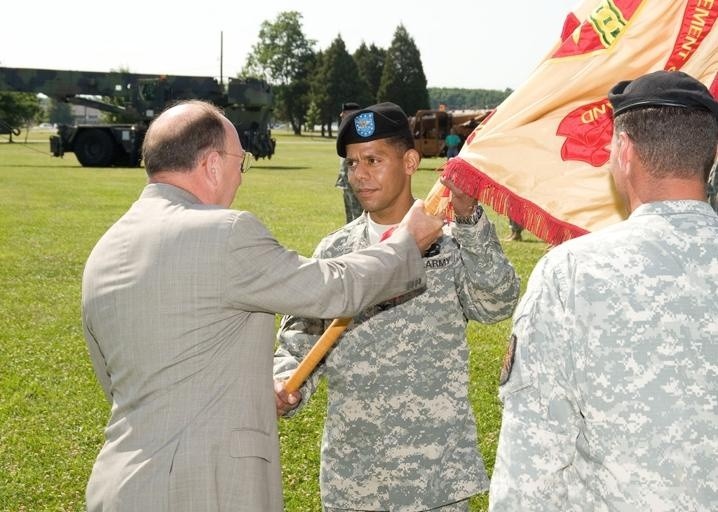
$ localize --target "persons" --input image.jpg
[487,69,718,512]
[446,127,461,161]
[504,218,525,242]
[333,101,363,225]
[80,97,445,512]
[271,102,520,512]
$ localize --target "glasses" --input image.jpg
[216,150,253,173]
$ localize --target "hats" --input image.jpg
[607,71,718,124]
[336,102,408,159]
[342,103,361,110]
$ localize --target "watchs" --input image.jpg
[453,205,485,227]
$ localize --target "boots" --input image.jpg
[505,231,521,242]
[504,229,513,239]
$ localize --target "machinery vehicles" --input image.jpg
[49,75,277,168]
[408,109,486,157]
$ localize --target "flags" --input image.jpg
[377,0,718,253]
